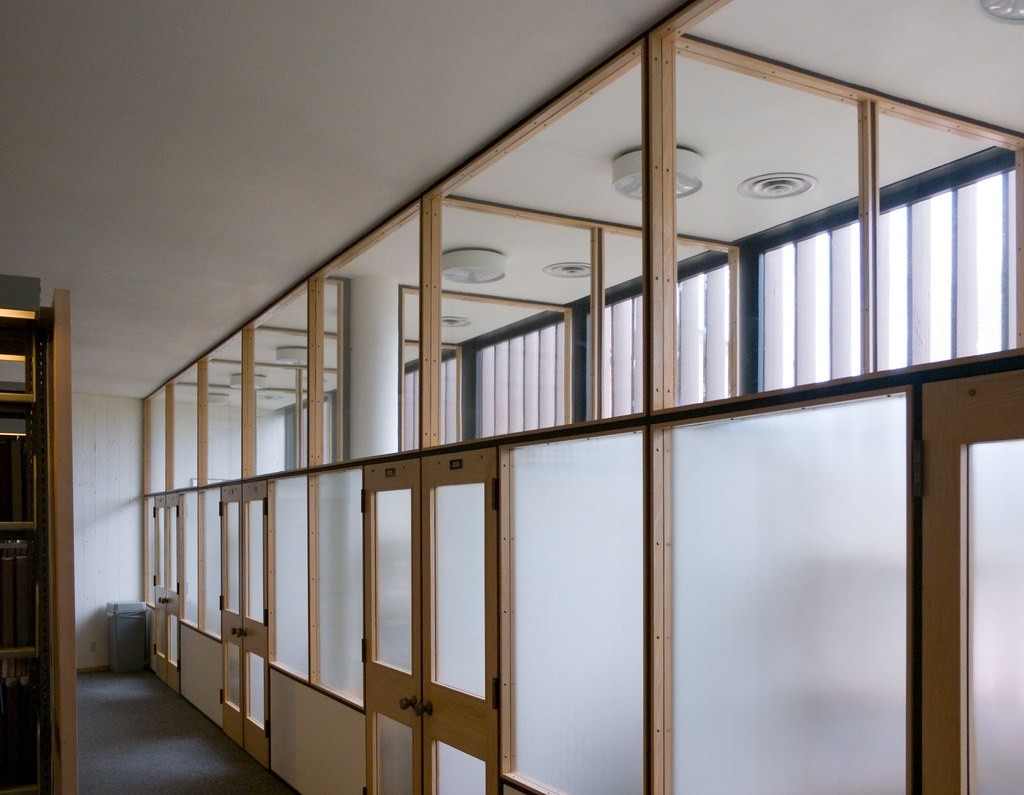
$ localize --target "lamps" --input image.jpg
[442,249,509,284]
[611,149,704,202]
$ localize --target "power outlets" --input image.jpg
[88,639,97,651]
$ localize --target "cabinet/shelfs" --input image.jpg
[0,288,79,795]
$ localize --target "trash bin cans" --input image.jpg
[104,600,148,674]
